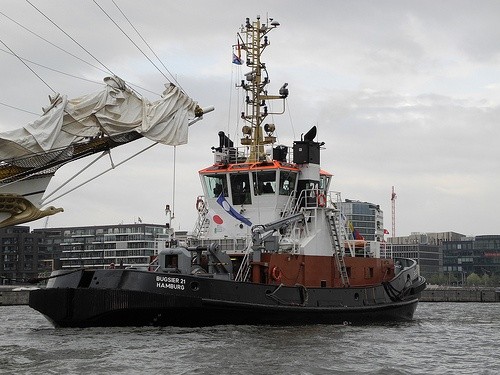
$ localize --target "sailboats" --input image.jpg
[0,0,215,228]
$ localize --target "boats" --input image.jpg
[29,14,427,331]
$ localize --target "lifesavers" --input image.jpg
[318,194,326,207]
[196,199,205,211]
[272,266,282,282]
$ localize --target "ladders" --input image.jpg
[327,215,350,287]
